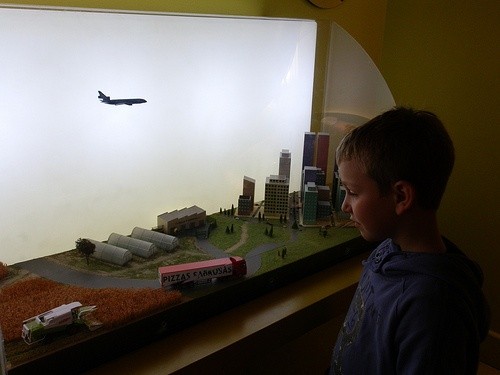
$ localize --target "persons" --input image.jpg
[330,106,492,375]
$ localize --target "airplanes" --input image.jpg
[97,91,147,105]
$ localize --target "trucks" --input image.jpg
[21,301,84,346]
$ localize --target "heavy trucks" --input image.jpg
[158,256,248,289]
